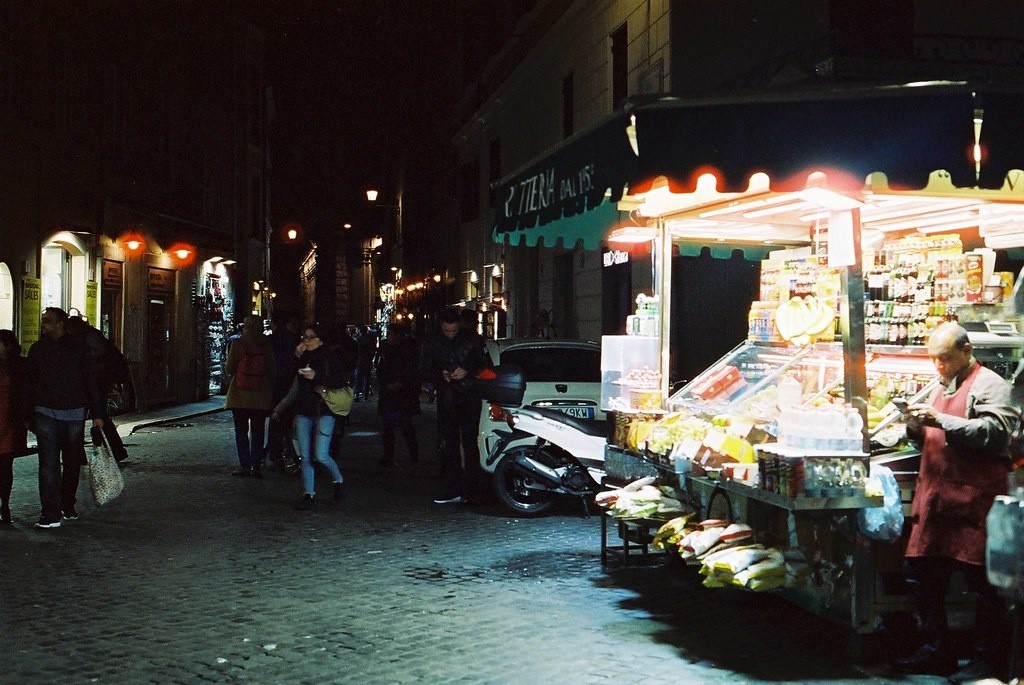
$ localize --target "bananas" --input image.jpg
[865,412,887,429]
[775,294,832,340]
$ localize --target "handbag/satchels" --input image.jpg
[83,425,125,507]
[314,386,355,417]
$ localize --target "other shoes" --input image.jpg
[112,448,128,462]
[60,505,79,520]
[1,506,11,524]
[949,655,1007,684]
[247,463,260,477]
[263,461,285,474]
[334,481,345,504]
[216,392,225,395]
[34,514,62,529]
[295,493,314,510]
[280,461,301,474]
[232,464,248,476]
[889,644,953,676]
[433,496,462,504]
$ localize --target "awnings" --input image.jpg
[492,73,976,262]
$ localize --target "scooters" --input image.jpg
[473,364,690,517]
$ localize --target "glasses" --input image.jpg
[300,334,319,342]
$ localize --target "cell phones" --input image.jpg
[892,398,910,410]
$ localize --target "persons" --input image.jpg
[889,322,1020,685]
[225,310,494,511]
[0,307,130,529]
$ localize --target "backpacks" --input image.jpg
[83,327,129,383]
[234,338,267,388]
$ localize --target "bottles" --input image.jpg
[985,487,1024,592]
[863,232,965,345]
[756,449,867,499]
[775,403,864,453]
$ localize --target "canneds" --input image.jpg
[933,258,950,301]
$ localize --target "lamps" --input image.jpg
[173,238,194,261]
[123,226,144,250]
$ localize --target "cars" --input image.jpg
[474,337,601,472]
